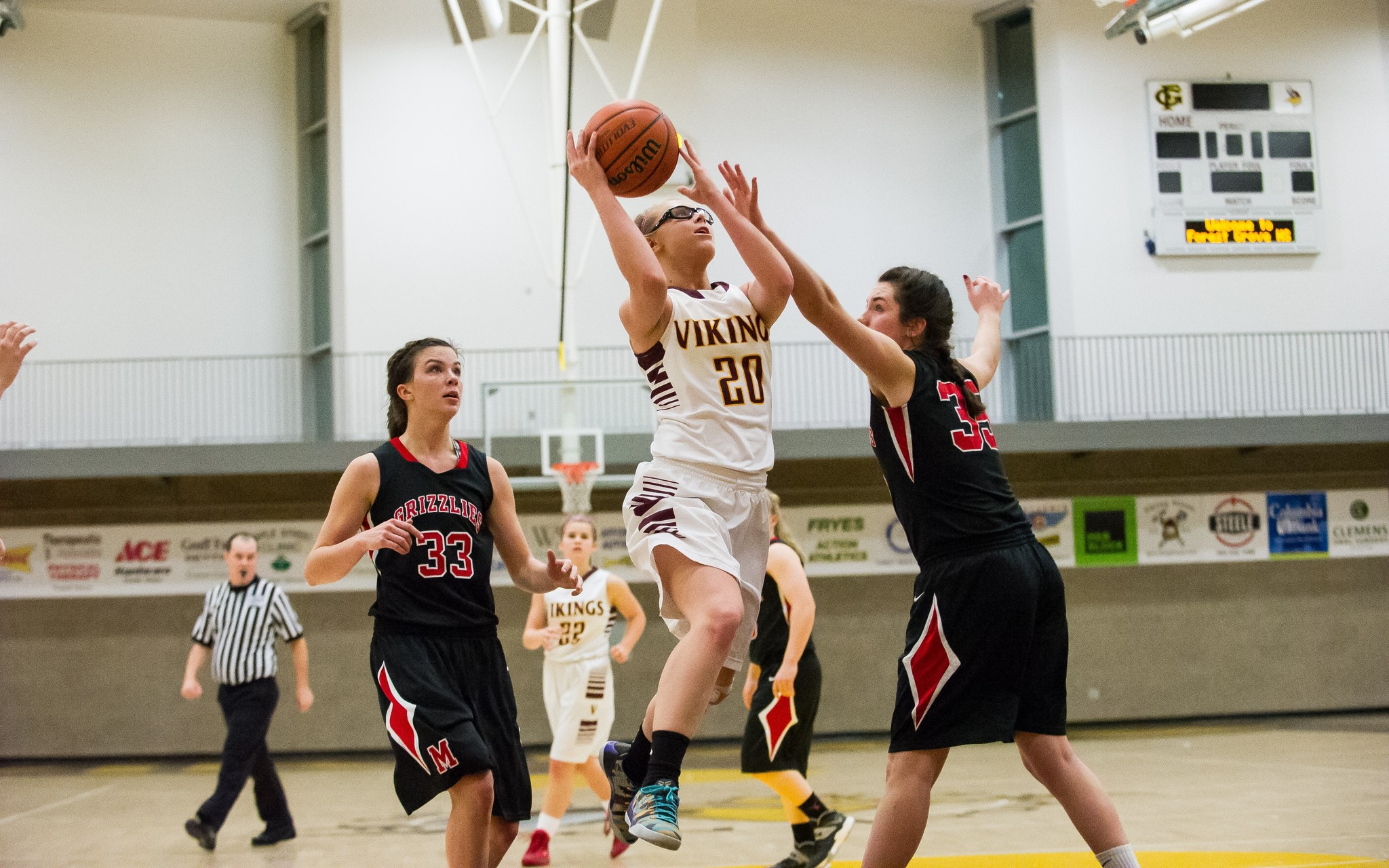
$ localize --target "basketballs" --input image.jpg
[583,99,679,199]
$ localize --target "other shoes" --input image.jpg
[251,820,296,845]
[185,818,217,851]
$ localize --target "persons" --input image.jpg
[717,162,1139,868]
[740,489,855,868]
[0,320,38,396]
[307,337,582,868]
[181,534,315,850]
[564,129,794,851]
[520,514,647,868]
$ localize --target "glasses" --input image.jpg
[650,206,714,234]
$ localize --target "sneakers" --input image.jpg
[625,778,682,851]
[805,810,855,868]
[521,829,550,866]
[767,839,816,868]
[603,808,629,858]
[598,741,639,845]
